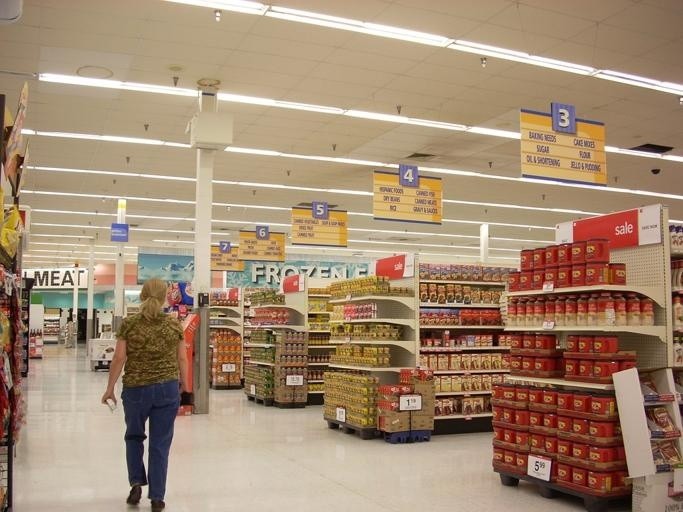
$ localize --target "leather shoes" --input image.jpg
[126,485,143,506]
[151,500,166,512]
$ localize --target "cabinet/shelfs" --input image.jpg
[0,92,61,512]
[88,339,118,372]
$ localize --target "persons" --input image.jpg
[100,278,189,512]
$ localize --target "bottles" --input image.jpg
[506,292,655,327]
[668,224,683,369]
[254,309,289,325]
[30,329,42,337]
